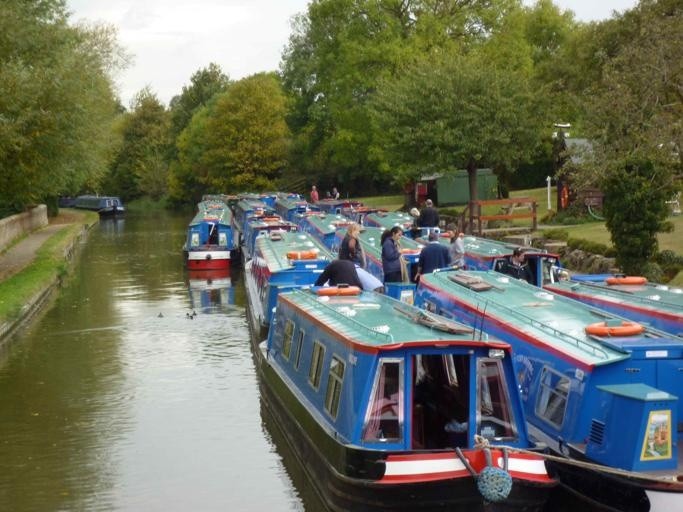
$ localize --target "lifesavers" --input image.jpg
[287,250,317,259]
[606,276,648,284]
[586,320,644,337]
[312,285,361,295]
[560,187,568,207]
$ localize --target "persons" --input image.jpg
[341,223,366,269]
[379,225,404,282]
[354,264,384,294]
[410,208,421,238]
[499,247,534,285]
[446,223,466,270]
[419,199,440,236]
[314,255,363,291]
[414,232,451,282]
[310,185,319,203]
[331,188,340,200]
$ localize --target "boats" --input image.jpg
[412,269,682,494]
[258,282,562,512]
[57,191,125,216]
[543,273,683,339]
[181,190,567,341]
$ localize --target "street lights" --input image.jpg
[551,123,571,214]
